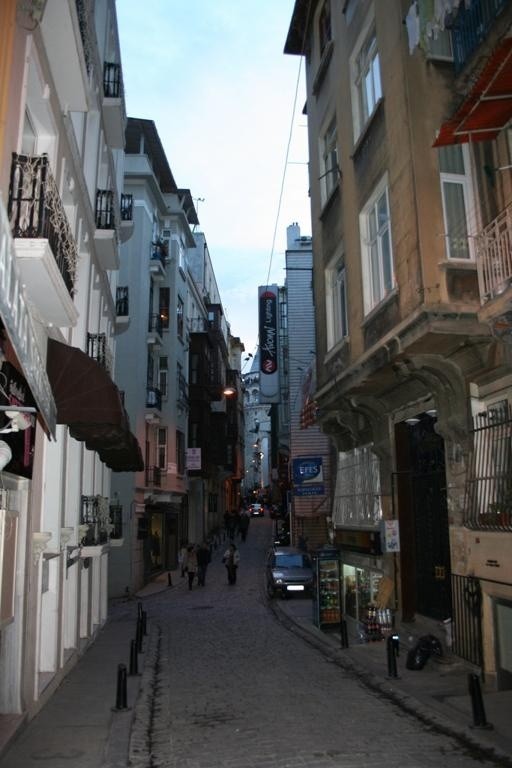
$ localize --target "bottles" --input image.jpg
[364,607,394,644]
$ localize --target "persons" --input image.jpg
[176,507,250,591]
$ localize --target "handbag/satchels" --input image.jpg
[184,566,188,572]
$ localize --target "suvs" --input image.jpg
[264,546,316,598]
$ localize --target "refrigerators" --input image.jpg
[312,548,343,634]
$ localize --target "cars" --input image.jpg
[248,502,265,516]
